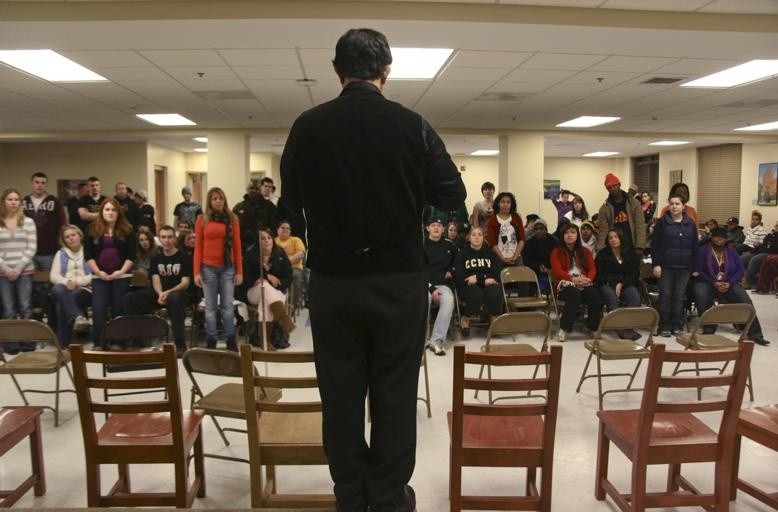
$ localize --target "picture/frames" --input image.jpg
[757,162,778,206]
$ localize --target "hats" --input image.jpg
[727,217,740,225]
[579,220,595,229]
[181,186,193,194]
[533,218,548,229]
[135,190,149,202]
[427,216,444,224]
[605,173,622,187]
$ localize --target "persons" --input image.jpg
[0,187,38,354]
[426,173,777,356]
[23,174,305,350]
[282,27,468,511]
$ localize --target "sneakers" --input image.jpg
[557,328,567,343]
[428,338,446,355]
[588,324,716,346]
[57,313,240,358]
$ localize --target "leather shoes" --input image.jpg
[460,320,471,334]
[754,336,770,346]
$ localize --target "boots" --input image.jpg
[269,301,297,333]
[258,321,277,351]
